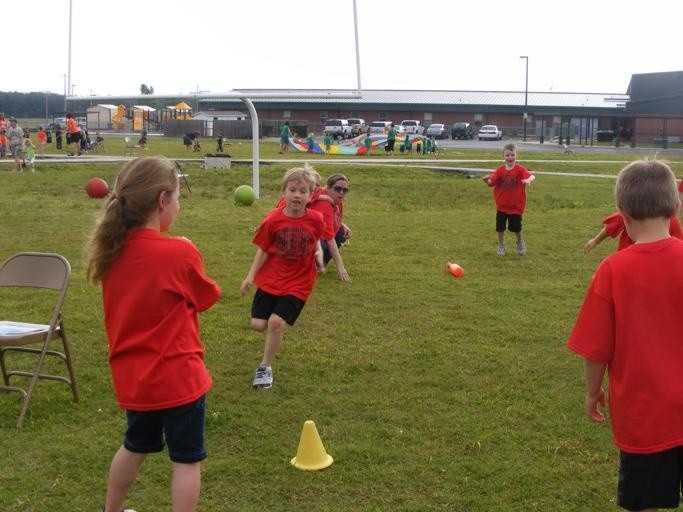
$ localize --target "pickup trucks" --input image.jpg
[395,119,425,137]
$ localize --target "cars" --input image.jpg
[479,124,502,141]
[426,123,450,139]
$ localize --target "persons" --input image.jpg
[23,138,36,173]
[584,209,683,254]
[274,163,335,275]
[142,131,148,144]
[357,128,361,136]
[55,127,62,150]
[0,128,8,158]
[46,127,52,144]
[324,133,333,154]
[279,121,293,154]
[7,119,24,172]
[216,128,224,152]
[404,135,411,153]
[387,123,401,154]
[85,155,222,512]
[430,135,435,153]
[239,166,326,390]
[566,159,682,512]
[306,132,314,153]
[482,144,535,256]
[0,112,8,128]
[312,173,352,281]
[77,127,87,151]
[183,131,200,152]
[365,134,372,157]
[36,127,47,159]
[64,112,81,157]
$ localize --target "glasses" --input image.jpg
[332,186,348,194]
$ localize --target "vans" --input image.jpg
[347,118,370,136]
[370,120,393,133]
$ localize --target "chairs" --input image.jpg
[124,136,141,155]
[562,143,574,157]
[173,160,192,193]
[0,251,81,426]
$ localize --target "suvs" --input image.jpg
[451,122,475,140]
[322,118,353,140]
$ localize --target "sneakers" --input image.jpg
[515,241,526,256]
[498,244,506,257]
[252,366,274,393]
